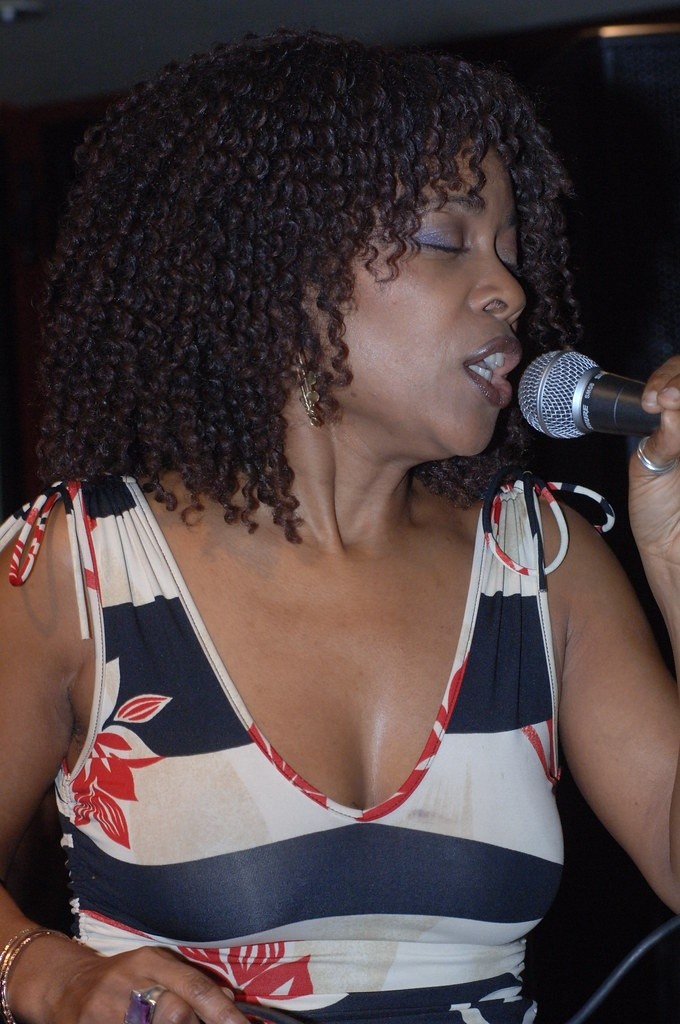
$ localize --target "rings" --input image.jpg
[637,436,676,473]
[122,984,168,1024]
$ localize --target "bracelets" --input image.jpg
[0,927,72,1024]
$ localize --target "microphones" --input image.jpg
[517,349,661,439]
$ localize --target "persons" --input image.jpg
[0,26,680,1024]
